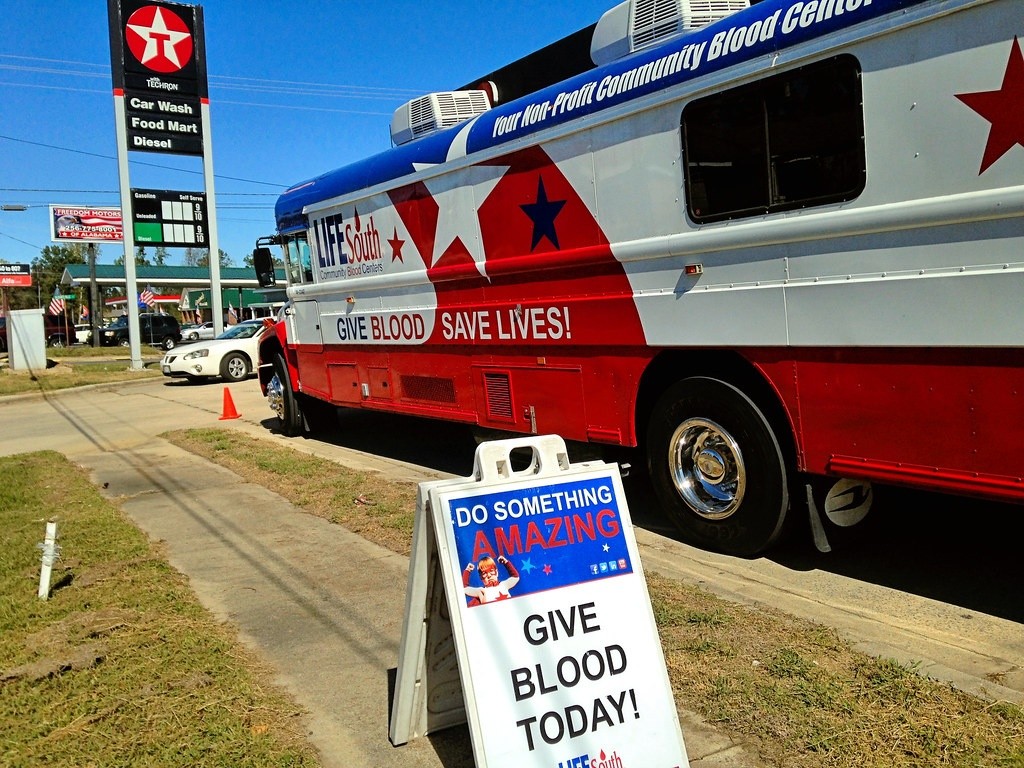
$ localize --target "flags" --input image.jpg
[49,286,65,316]
[138,286,157,310]
[227,302,239,321]
[195,304,202,326]
[80,304,89,320]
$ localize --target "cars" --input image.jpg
[75,324,101,344]
[181,322,228,341]
[160,316,278,384]
[0,314,75,351]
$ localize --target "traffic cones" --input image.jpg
[219,388,242,420]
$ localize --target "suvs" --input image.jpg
[98,313,183,351]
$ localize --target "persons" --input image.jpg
[460,556,522,607]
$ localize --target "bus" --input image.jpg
[252,1,1024,558]
